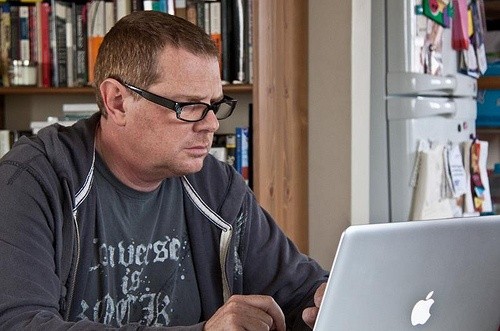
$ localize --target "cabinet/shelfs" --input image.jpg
[0,0,309,257]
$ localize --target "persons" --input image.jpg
[0,11,330,331]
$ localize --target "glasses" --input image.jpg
[108,76,238,123]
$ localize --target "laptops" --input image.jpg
[312,214,500,331]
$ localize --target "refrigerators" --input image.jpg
[370,0,482,223]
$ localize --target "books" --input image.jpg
[208,104,254,190]
[0,0,253,88]
[0,103,100,158]
[413,140,492,221]
